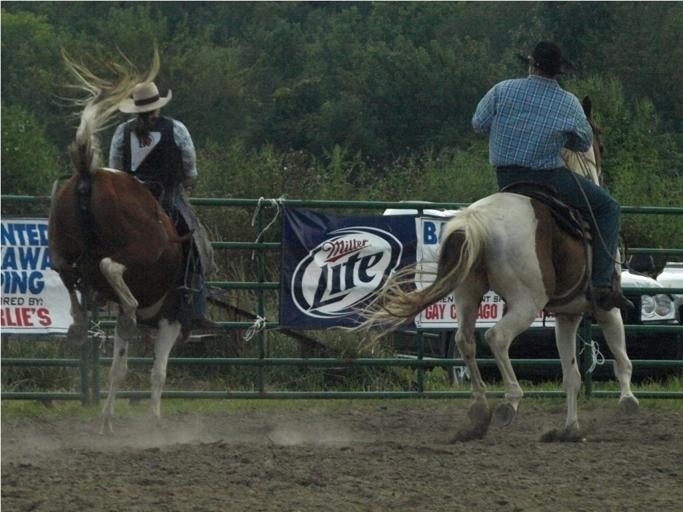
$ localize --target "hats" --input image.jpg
[117,82,173,115]
[516,39,575,79]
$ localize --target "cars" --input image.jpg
[45,318,220,390]
[359,264,682,384]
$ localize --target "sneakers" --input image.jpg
[599,286,634,311]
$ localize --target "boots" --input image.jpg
[181,273,220,328]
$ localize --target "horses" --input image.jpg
[327,93,642,444]
[46,37,204,438]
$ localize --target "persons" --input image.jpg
[106,81,220,330]
[471,38,630,307]
